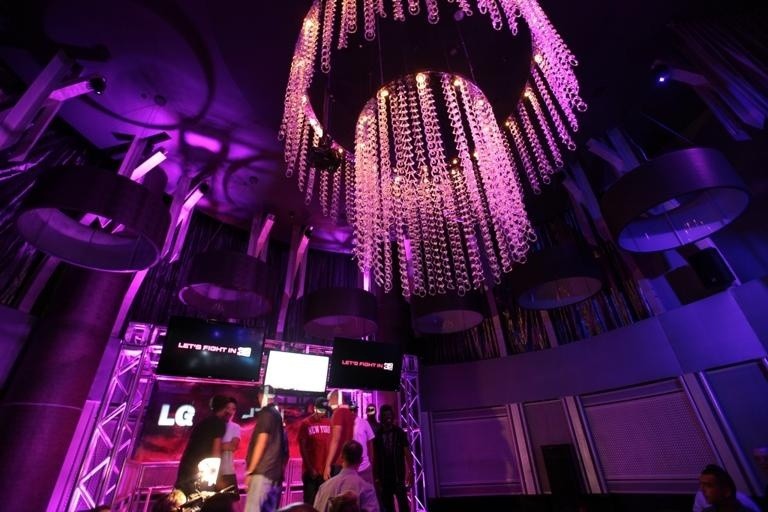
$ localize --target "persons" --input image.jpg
[312,440,381,512]
[296,396,332,504]
[172,394,227,493]
[214,396,242,493]
[373,404,414,512]
[691,462,761,511]
[243,384,291,511]
[323,388,356,482]
[347,399,377,490]
[157,487,188,512]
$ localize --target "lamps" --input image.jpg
[0,0,755,345]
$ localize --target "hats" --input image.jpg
[313,397,332,413]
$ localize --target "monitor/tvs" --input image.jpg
[263,348,330,396]
[155,314,267,382]
[328,336,405,393]
